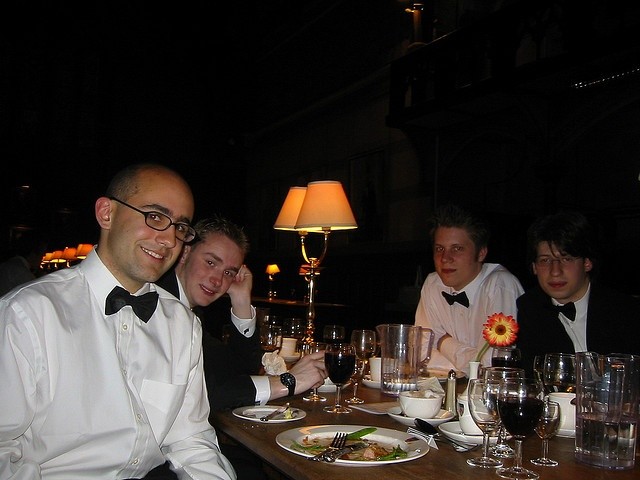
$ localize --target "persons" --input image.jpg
[153,215,332,480]
[0,158,237,480]
[408,211,526,381]
[516,222,639,400]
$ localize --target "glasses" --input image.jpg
[538,255,585,264]
[109,196,198,242]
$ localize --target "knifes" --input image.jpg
[260,403,290,421]
[322,442,370,463]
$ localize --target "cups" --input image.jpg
[543,392,577,430]
[457,395,494,436]
[277,336,298,357]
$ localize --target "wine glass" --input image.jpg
[533,355,545,386]
[496,377,544,480]
[543,351,578,393]
[491,347,522,369]
[345,355,365,404]
[322,344,356,415]
[302,342,328,402]
[323,325,345,344]
[350,329,377,385]
[481,366,525,459]
[530,401,559,467]
[466,379,503,469]
[258,313,306,353]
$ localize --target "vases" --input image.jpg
[456,362,490,436]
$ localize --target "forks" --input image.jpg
[306,432,348,461]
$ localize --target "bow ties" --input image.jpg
[104,286,159,323]
[553,302,576,321]
[442,291,469,308]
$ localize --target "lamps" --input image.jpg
[265,263,281,301]
[60,247,78,268]
[299,264,321,304]
[40,250,53,272]
[74,244,94,260]
[49,250,66,270]
[272,180,358,351]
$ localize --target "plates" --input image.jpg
[231,403,308,423]
[275,424,430,468]
[437,421,513,447]
[277,352,301,363]
[317,377,351,393]
[387,406,455,429]
[421,366,465,384]
[362,374,417,392]
[556,428,577,439]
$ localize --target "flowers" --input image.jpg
[474,311,519,361]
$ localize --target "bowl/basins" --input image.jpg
[369,357,397,381]
[398,392,444,418]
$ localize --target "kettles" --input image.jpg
[573,355,640,471]
[375,324,435,398]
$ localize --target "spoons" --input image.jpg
[414,417,475,451]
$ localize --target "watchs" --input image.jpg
[282,374,295,397]
